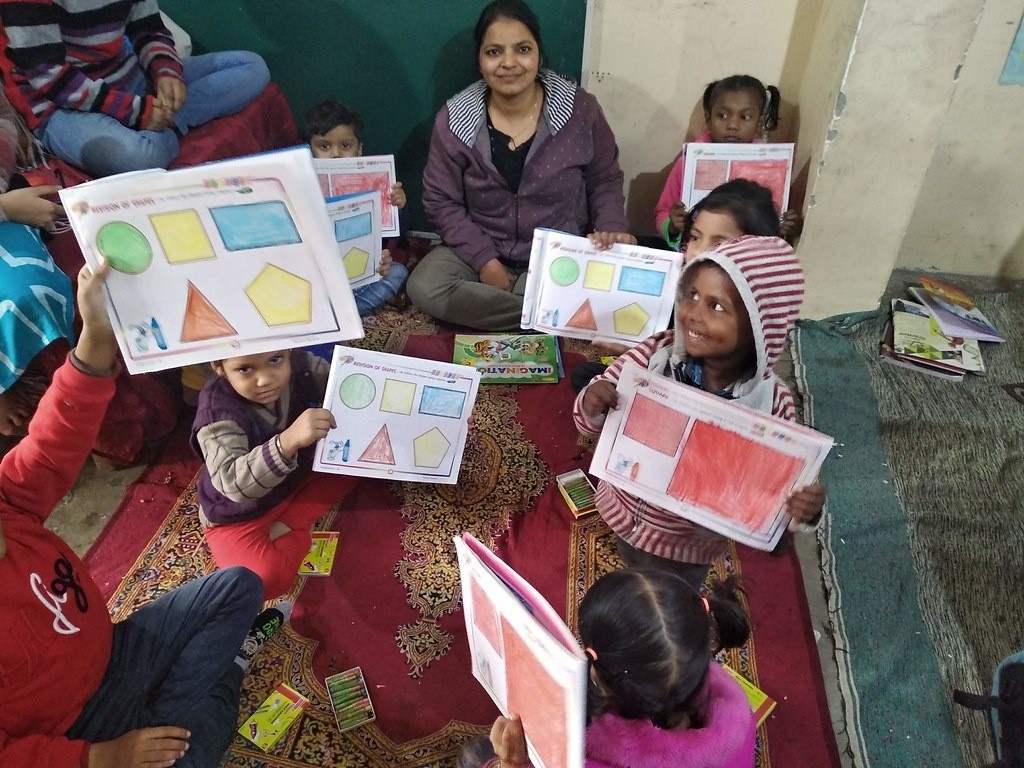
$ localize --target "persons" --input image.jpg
[406,0,638,339]
[653,74,799,256]
[571,177,782,394]
[0,254,290,768]
[571,235,827,599]
[0,89,75,435]
[483,567,757,768]
[188,348,361,601]
[301,99,408,315]
[0,0,272,176]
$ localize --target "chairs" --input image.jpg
[0,38,302,471]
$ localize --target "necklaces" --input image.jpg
[489,91,538,139]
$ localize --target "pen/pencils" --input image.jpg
[54,168,66,189]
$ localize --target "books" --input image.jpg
[446,533,588,768]
[880,273,1007,384]
[589,362,835,553]
[51,149,366,378]
[313,346,481,484]
[681,142,793,222]
[326,190,383,289]
[313,154,400,237]
[521,227,685,349]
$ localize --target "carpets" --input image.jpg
[79,236,843,768]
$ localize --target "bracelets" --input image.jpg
[70,347,118,376]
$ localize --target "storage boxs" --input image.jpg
[296,531,341,577]
[721,664,777,730]
[556,468,600,519]
[238,680,311,755]
